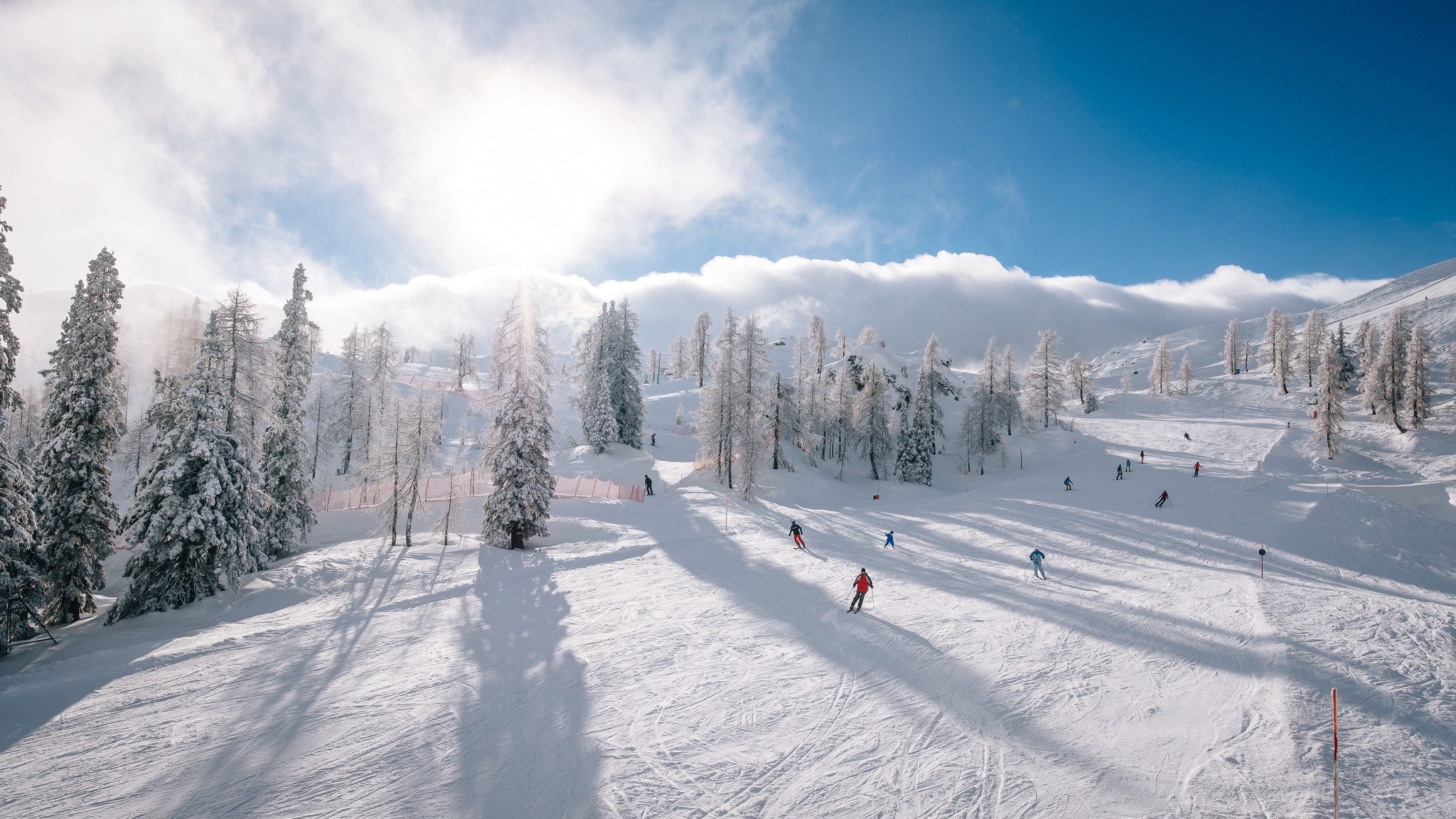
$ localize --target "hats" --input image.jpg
[792,521,795,525]
[1127,459,1129,460]
[1164,490,1166,492]
[1197,462,1199,463]
[1119,464,1121,466]
[645,475,647,477]
[861,568,865,572]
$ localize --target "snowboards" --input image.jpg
[1122,471,1133,472]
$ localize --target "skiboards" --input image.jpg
[846,606,862,614]
[1154,504,1163,509]
[882,546,896,549]
[1034,574,1047,580]
[1113,478,1126,480]
[1192,476,1199,477]
[650,444,660,447]
[794,546,808,550]
[1063,489,1074,491]
[1137,463,1147,464]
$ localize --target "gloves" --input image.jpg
[789,534,790,536]
[1031,558,1033,560]
[1042,557,1044,559]
[801,532,803,535]
[871,586,873,588]
[853,584,856,587]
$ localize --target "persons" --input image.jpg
[1155,490,1169,507]
[884,531,894,548]
[1064,476,1073,490]
[1184,432,1192,441]
[788,519,805,548]
[1140,450,1145,463]
[846,568,874,609]
[1029,546,1045,579]
[651,433,656,446]
[1193,462,1203,476]
[1125,459,1131,472]
[644,475,653,495]
[1116,464,1123,480]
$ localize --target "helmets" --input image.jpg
[1034,546,1039,549]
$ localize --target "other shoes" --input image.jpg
[1129,470,1130,471]
[651,493,653,495]
[1067,489,1069,490]
[647,493,650,495]
[1070,489,1072,490]
[1121,478,1123,479]
[803,546,805,548]
[798,546,802,548]
[1125,470,1127,472]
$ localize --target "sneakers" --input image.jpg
[857,607,860,610]
[1035,575,1038,577]
[1042,575,1045,578]
[849,605,853,609]
